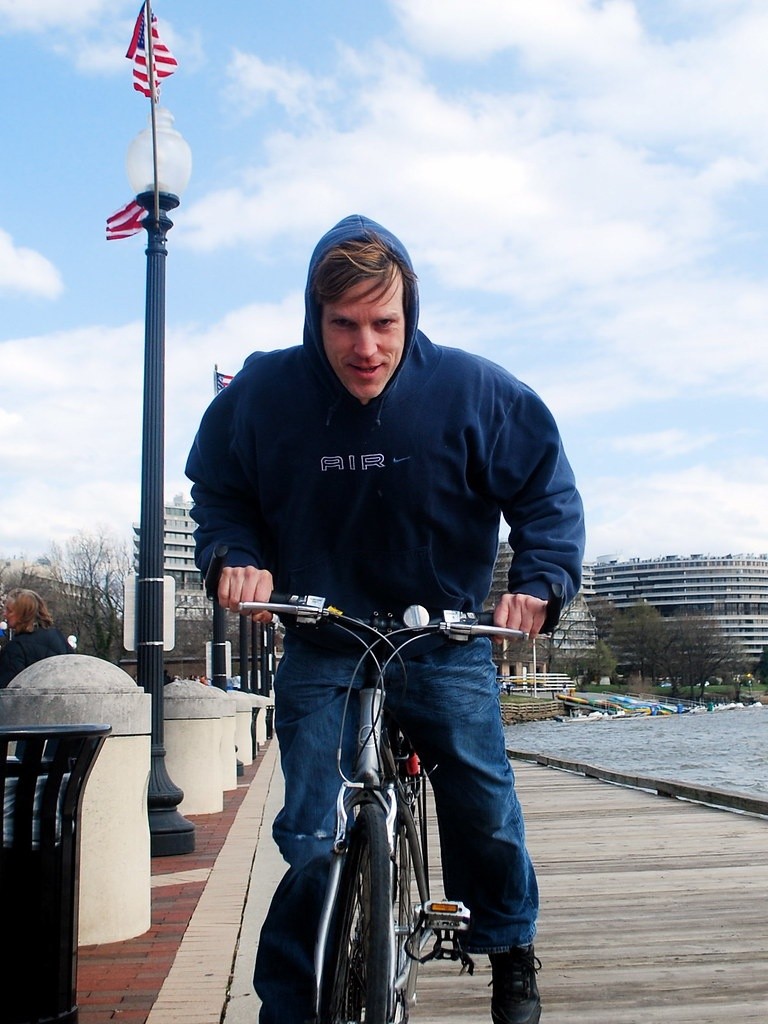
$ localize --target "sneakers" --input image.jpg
[487,942,543,1024]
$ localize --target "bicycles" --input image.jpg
[233,592,533,1024]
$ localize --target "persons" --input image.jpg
[0,587,76,690]
[186,213,584,1024]
[190,675,208,685]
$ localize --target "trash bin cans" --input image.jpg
[266,705,275,740]
[251,707,261,759]
[0,724,114,1024]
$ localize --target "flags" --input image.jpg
[217,373,233,390]
[106,200,149,240]
[125,0,176,99]
